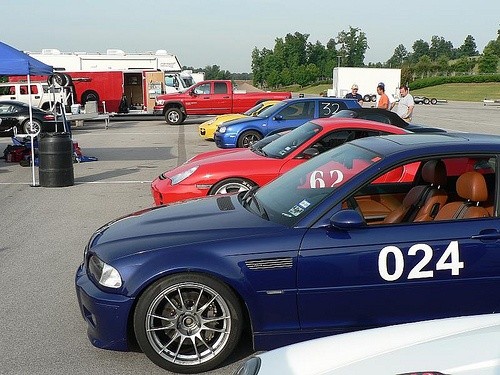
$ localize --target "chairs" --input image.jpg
[383,160,448,223]
[343,131,360,143]
[433,172,490,221]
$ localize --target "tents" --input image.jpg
[0,42,57,187]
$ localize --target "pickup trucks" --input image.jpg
[0,82,69,114]
[155,79,292,125]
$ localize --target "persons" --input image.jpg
[377,85,390,109]
[346,84,364,105]
[397,86,415,123]
[377,82,395,109]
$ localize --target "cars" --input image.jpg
[0,100,71,137]
[148,117,416,208]
[326,108,448,135]
[72,131,500,375]
[214,97,364,149]
[234,311,500,375]
[197,99,311,142]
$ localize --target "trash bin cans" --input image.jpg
[36,131,75,188]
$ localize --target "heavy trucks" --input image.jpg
[319,67,448,105]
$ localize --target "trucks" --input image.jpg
[8,47,199,118]
